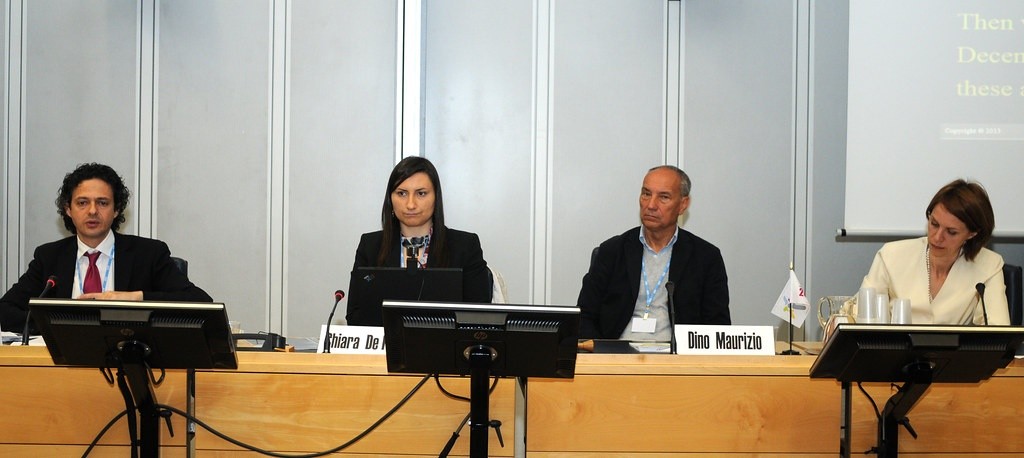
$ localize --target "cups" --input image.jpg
[874,289,891,323]
[854,287,877,323]
[891,298,912,325]
[229,321,241,347]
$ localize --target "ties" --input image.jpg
[82,251,102,294]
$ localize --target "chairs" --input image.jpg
[1003,262,1022,328]
[172,257,188,276]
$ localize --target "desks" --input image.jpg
[0,340,1024,458]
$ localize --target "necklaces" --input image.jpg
[927,249,931,304]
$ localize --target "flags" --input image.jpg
[772,270,811,328]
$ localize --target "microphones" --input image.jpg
[22,276,59,345]
[976,283,988,326]
[664,281,678,355]
[324,291,345,353]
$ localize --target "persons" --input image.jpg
[345,156,493,326]
[0,163,213,334]
[840,180,1010,326]
[575,166,731,341]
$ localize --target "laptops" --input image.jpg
[359,267,462,327]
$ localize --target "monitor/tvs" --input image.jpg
[382,300,581,378]
[809,323,1024,383]
[27,297,239,369]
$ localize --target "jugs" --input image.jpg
[816,296,856,348]
